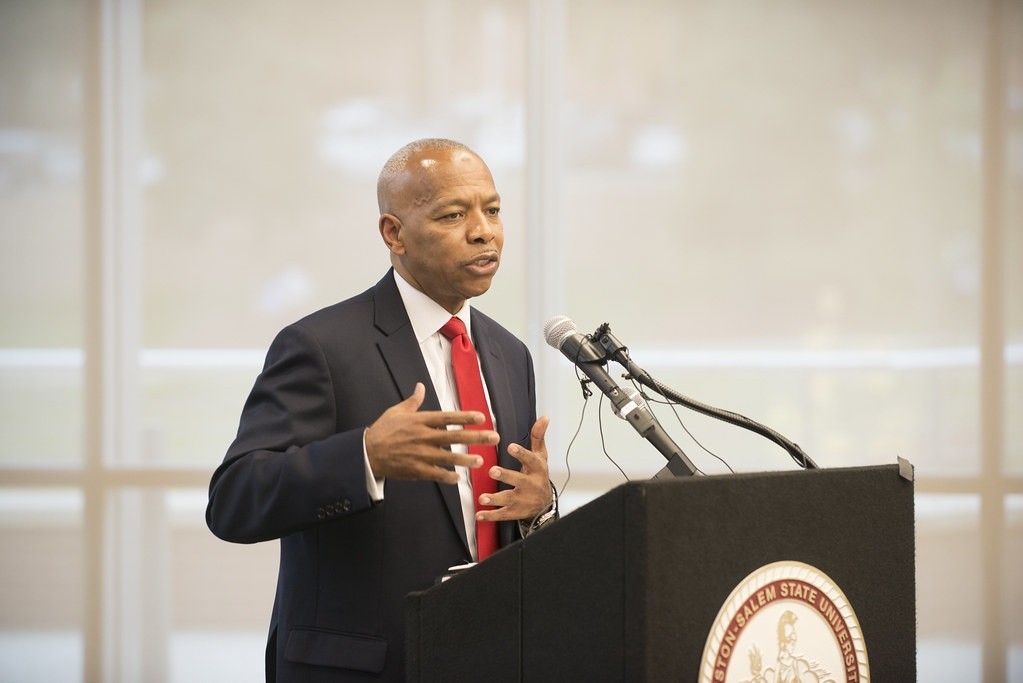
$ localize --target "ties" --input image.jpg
[439,317,499,562]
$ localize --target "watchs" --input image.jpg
[522,478,558,529]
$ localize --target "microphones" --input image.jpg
[542,315,656,438]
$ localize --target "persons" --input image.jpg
[205,138,551,683]
